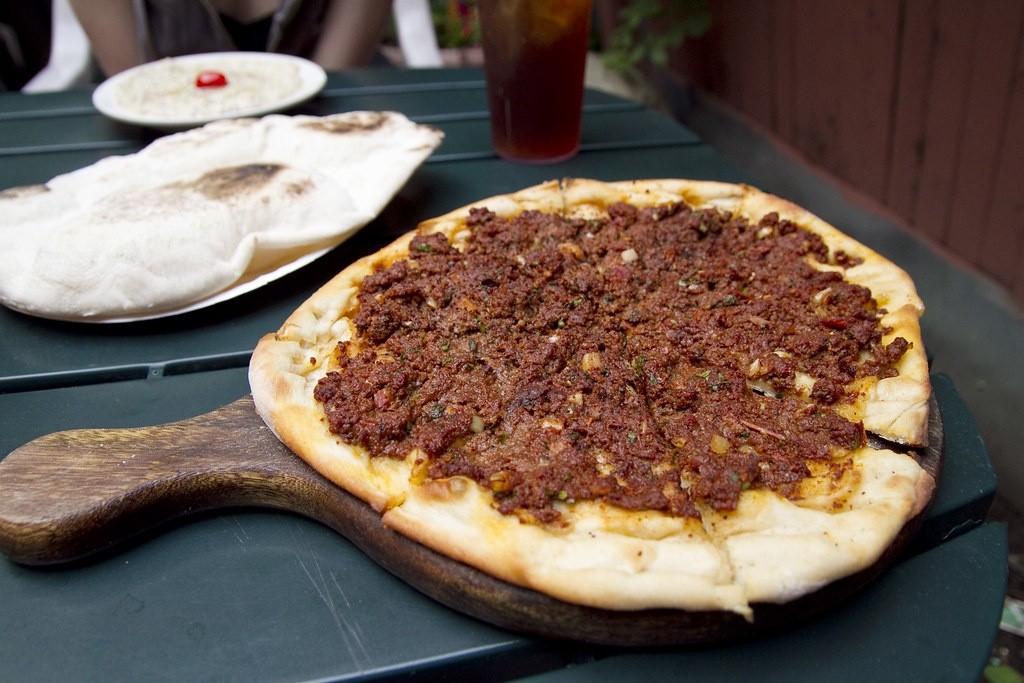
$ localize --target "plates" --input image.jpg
[91,52,328,132]
[0,244,339,323]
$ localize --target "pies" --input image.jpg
[117,57,303,124]
[0,110,444,319]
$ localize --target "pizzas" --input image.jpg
[248,174,937,621]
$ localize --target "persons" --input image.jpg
[67,0,392,78]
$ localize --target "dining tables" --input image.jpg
[1,60,1023,682]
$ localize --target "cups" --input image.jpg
[480,0,592,165]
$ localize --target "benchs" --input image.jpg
[653,78,1021,530]
